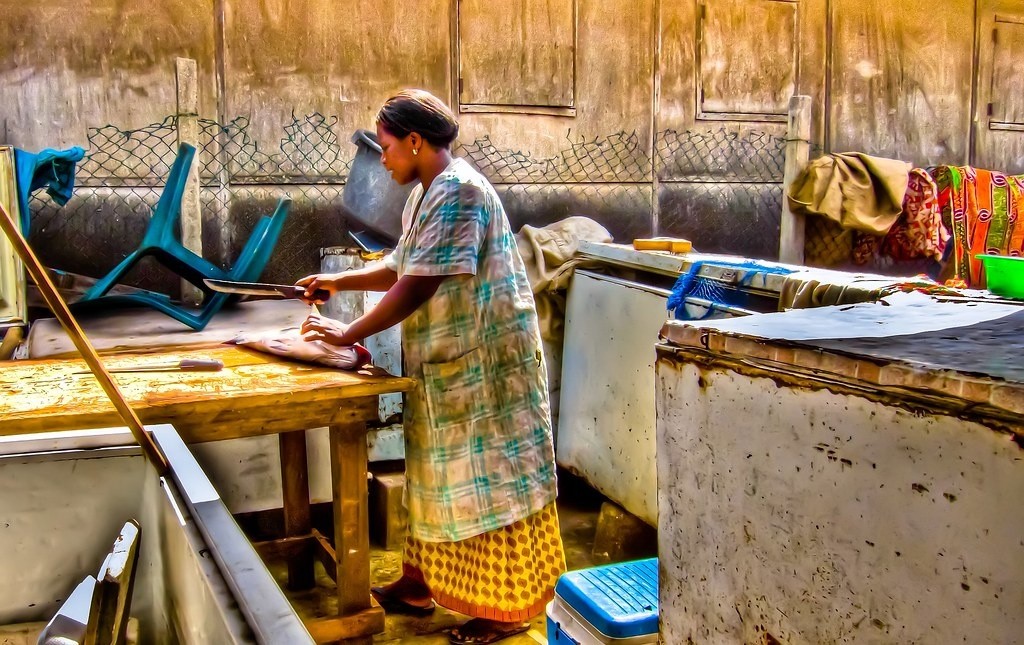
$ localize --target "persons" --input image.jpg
[292,88,567,645]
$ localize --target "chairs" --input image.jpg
[37,518,143,645]
[77,140,291,331]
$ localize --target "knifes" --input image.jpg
[73,360,224,374]
[204,277,330,303]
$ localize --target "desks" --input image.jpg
[0,340,416,645]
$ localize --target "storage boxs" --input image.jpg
[545,557,658,645]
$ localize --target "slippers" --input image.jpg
[447,619,531,645]
[371,586,435,616]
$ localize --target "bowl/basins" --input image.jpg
[338,129,421,247]
[975,254,1024,298]
